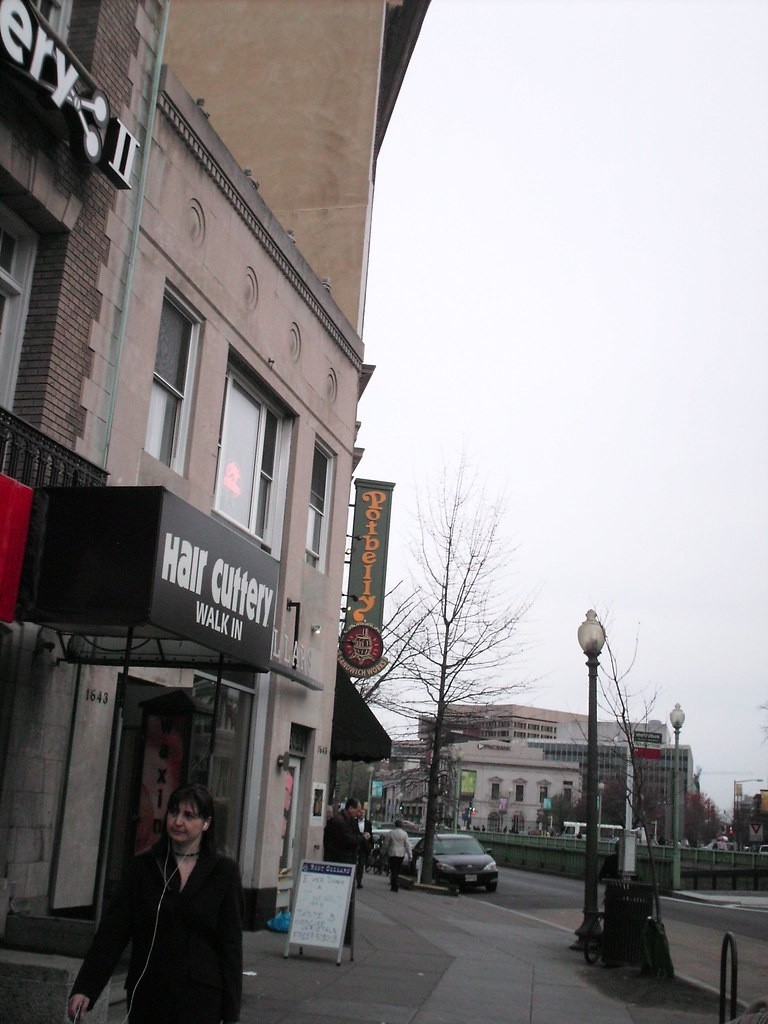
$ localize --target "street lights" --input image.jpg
[597,782,604,841]
[733,779,764,823]
[570,609,607,952]
[668,703,686,892]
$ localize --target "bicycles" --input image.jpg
[582,871,643,966]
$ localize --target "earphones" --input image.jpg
[203,821,208,829]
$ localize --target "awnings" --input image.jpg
[330,660,392,765]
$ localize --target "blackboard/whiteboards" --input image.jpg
[283,859,357,949]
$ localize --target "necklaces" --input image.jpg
[175,852,199,856]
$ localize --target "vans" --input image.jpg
[758,844,768,855]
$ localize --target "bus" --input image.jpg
[561,821,623,843]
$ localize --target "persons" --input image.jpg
[658,835,665,845]
[354,810,374,889]
[718,838,727,850]
[528,827,531,835]
[482,825,485,831]
[381,820,412,892]
[503,826,507,833]
[322,798,371,864]
[67,784,243,1024]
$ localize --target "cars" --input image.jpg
[336,801,451,879]
[405,834,499,896]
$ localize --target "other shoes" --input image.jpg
[357,885,363,889]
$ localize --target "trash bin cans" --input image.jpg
[602,877,661,969]
